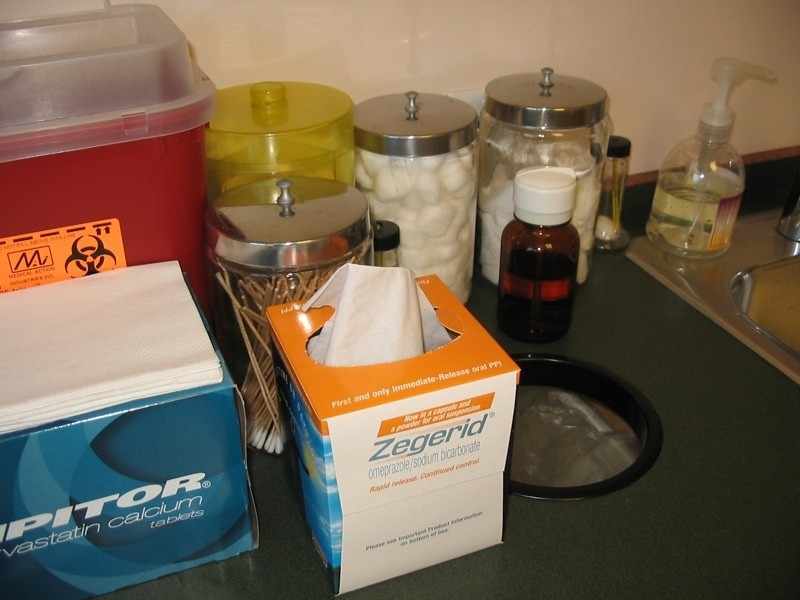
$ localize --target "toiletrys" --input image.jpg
[645,52,781,262]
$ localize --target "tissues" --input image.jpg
[265,262,522,600]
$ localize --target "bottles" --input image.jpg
[209,173,404,458]
[495,162,582,343]
[593,134,633,249]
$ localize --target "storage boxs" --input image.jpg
[1,274,264,599]
[259,271,523,596]
[1,2,222,338]
